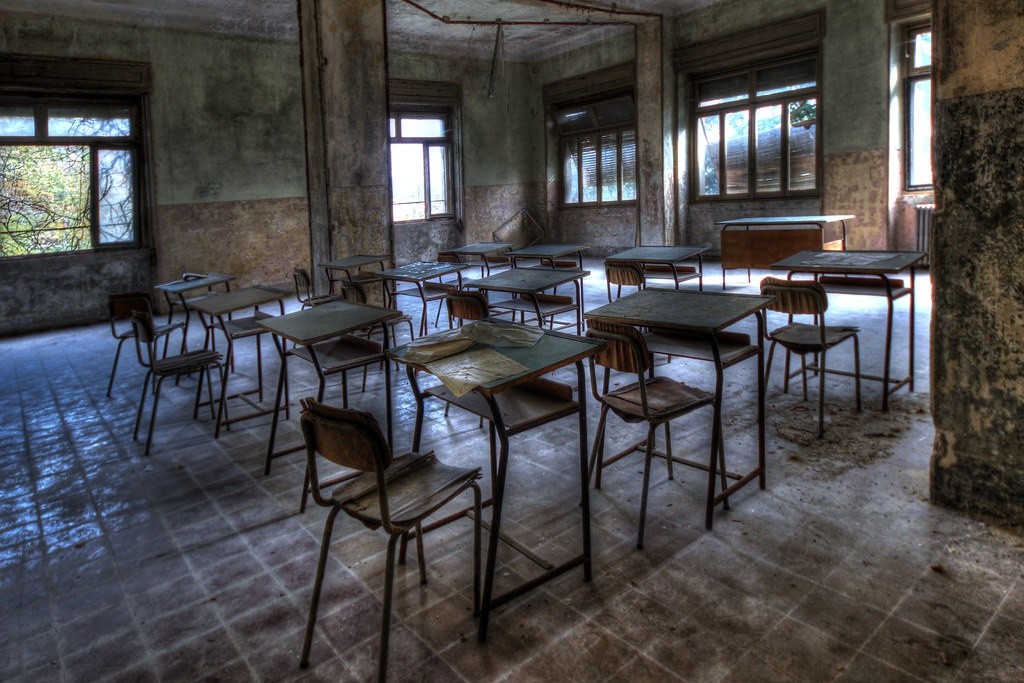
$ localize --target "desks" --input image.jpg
[464,266,591,335]
[604,245,710,298]
[448,242,515,301]
[317,254,389,335]
[186,287,290,439]
[385,317,610,644]
[770,249,929,413]
[580,287,776,530]
[714,215,855,289]
[254,302,403,514]
[155,273,237,386]
[378,260,472,338]
[504,243,593,331]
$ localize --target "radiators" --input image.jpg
[914,204,934,266]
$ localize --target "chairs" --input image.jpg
[435,252,480,328]
[292,268,353,349]
[445,289,489,428]
[106,293,190,397]
[579,317,730,549]
[758,277,863,439]
[340,280,414,392]
[603,259,672,364]
[130,310,230,456]
[299,396,483,683]
